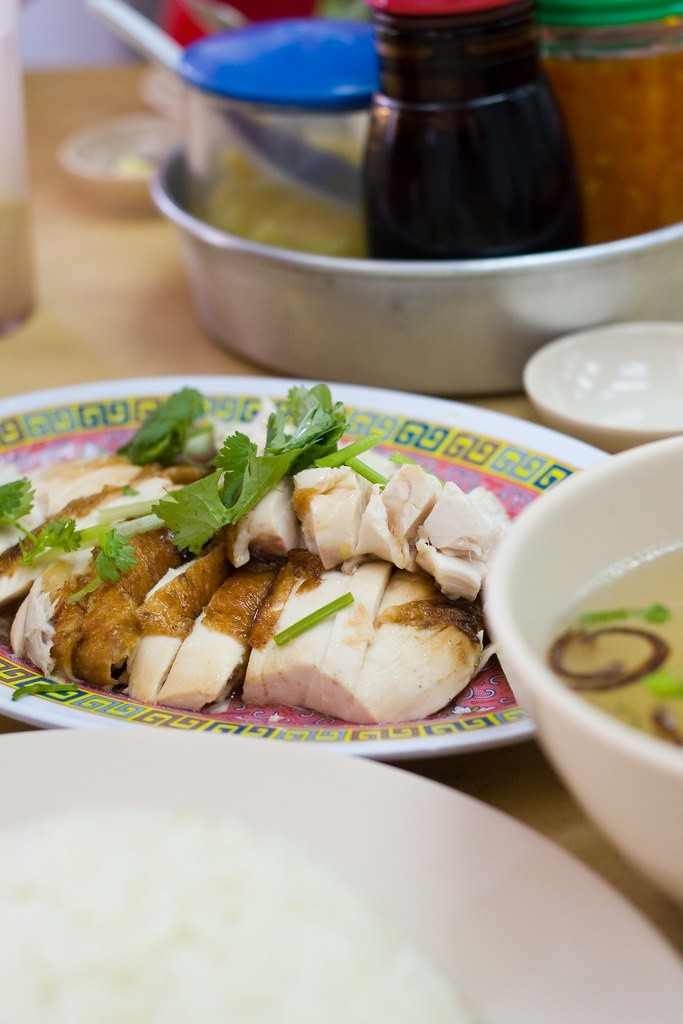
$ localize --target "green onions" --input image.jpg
[578,601,683,697]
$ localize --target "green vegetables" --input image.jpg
[0,379,390,643]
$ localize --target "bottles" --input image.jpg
[364,0,587,263]
[535,0,682,246]
[180,18,378,261]
[0,2,35,321]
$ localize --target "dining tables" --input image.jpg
[3,58,683,954]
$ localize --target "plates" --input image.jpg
[1,727,683,1024]
[523,320,683,453]
[0,373,611,762]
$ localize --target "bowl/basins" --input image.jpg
[60,123,181,208]
[486,436,682,911]
[151,146,682,397]
